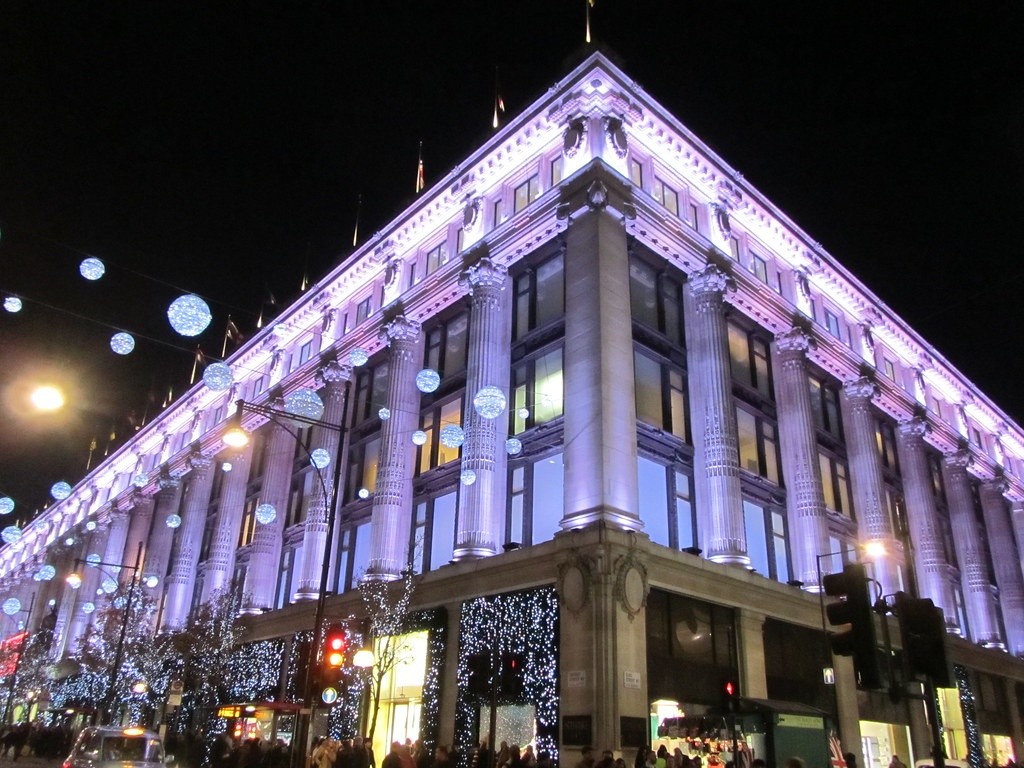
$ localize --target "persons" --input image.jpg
[1,722,753,768]
[888,755,907,768]
[985,758,1024,768]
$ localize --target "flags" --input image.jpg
[828,737,848,768]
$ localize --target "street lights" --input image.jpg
[224,379,354,767]
[67,540,144,724]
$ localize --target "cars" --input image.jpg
[60,724,174,768]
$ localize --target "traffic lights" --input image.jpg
[324,628,345,669]
[723,679,737,699]
[823,563,878,664]
